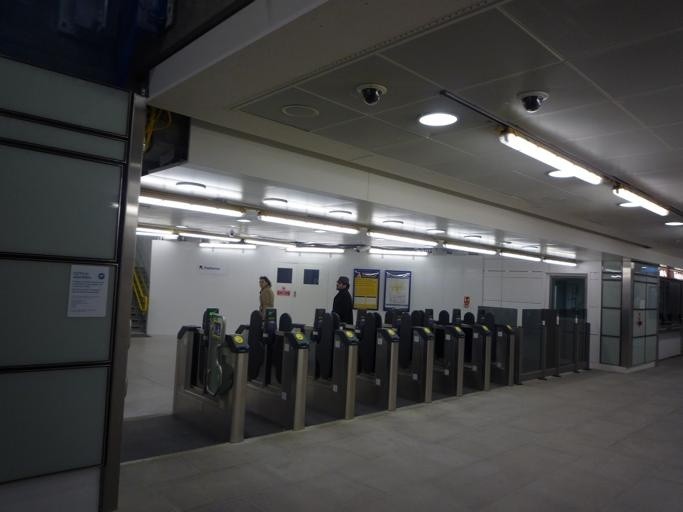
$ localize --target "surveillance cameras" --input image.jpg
[355,83,387,106]
[518,91,549,113]
[356,245,371,253]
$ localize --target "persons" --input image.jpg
[259,277,275,326]
[333,277,353,326]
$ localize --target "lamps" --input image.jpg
[611,182,670,219]
[498,125,603,186]
[133,191,578,269]
